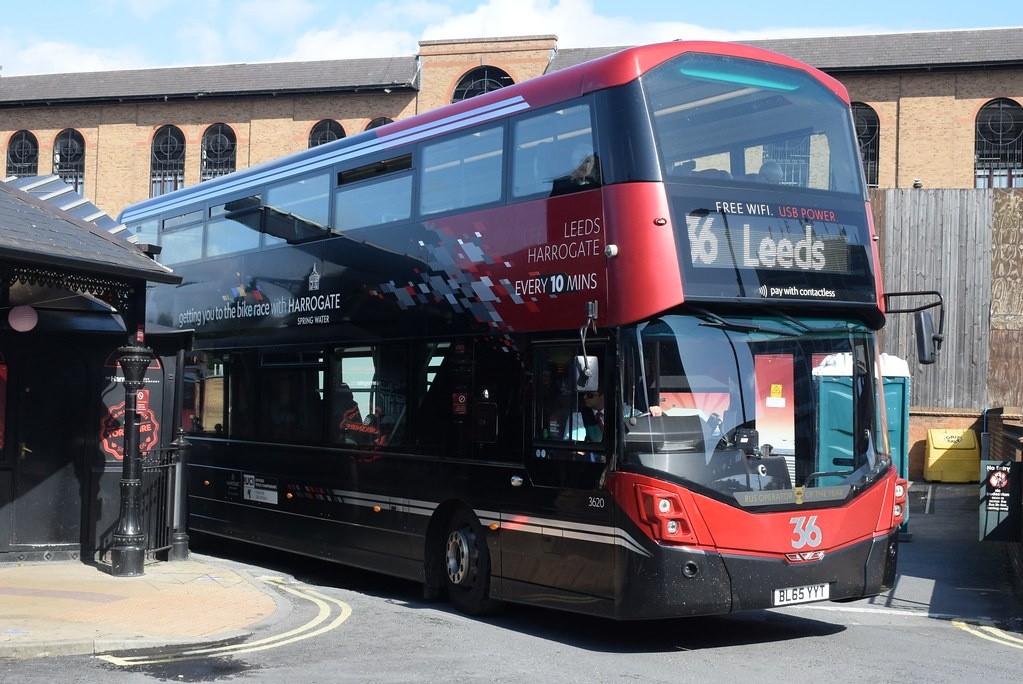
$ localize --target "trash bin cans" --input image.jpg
[924,429,981,483]
[813,352,912,542]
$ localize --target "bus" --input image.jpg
[116,40,948,638]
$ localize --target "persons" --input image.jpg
[550,142,602,196]
[562,391,662,443]
[758,160,782,184]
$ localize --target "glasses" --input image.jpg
[582,391,601,399]
[762,173,782,185]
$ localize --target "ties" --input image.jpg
[595,412,605,431]
